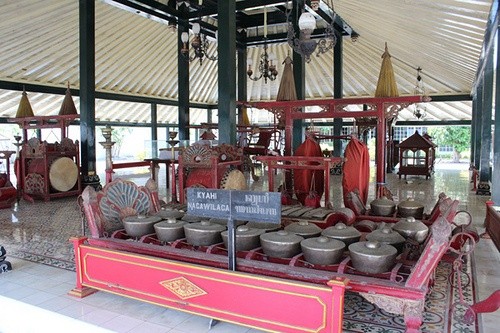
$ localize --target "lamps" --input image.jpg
[180,0,219,66]
[411,67,427,120]
[247,5,278,84]
[284,0,337,64]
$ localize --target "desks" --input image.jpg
[484,200,500,253]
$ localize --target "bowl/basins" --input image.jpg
[121,197,426,274]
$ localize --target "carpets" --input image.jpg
[0,194,484,333]
